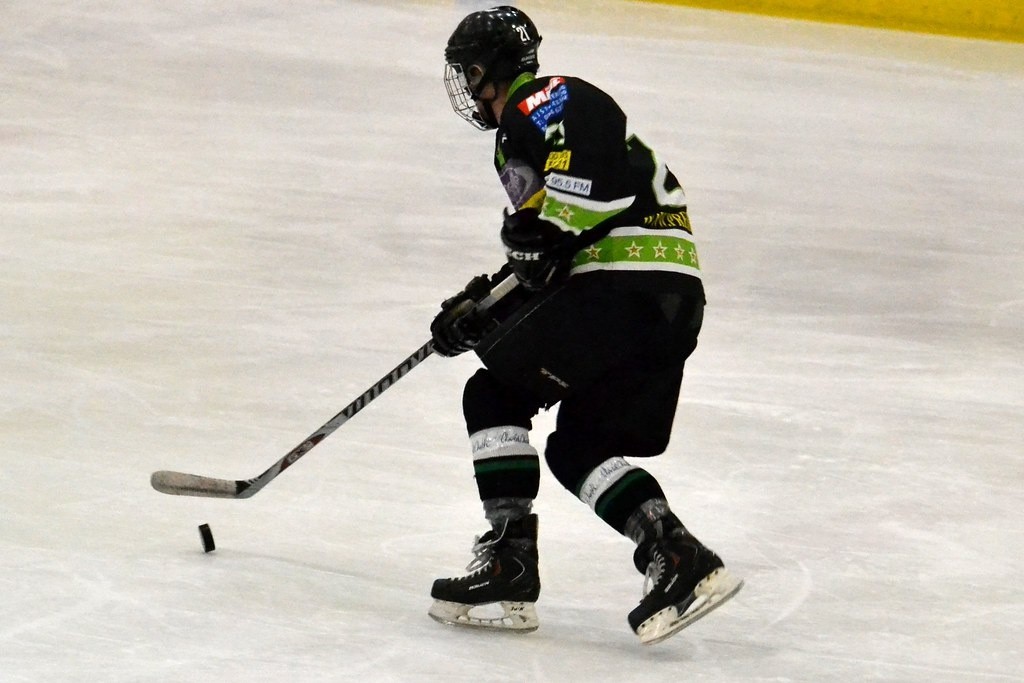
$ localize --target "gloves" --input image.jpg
[431,275,516,356]
[501,207,577,300]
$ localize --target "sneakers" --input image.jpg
[627,507,744,646]
[428,514,540,634]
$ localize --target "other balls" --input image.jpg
[196,523,217,553]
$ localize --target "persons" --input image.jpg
[428,6,745,643]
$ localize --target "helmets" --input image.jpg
[443,6,542,131]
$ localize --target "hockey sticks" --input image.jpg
[149,269,519,501]
